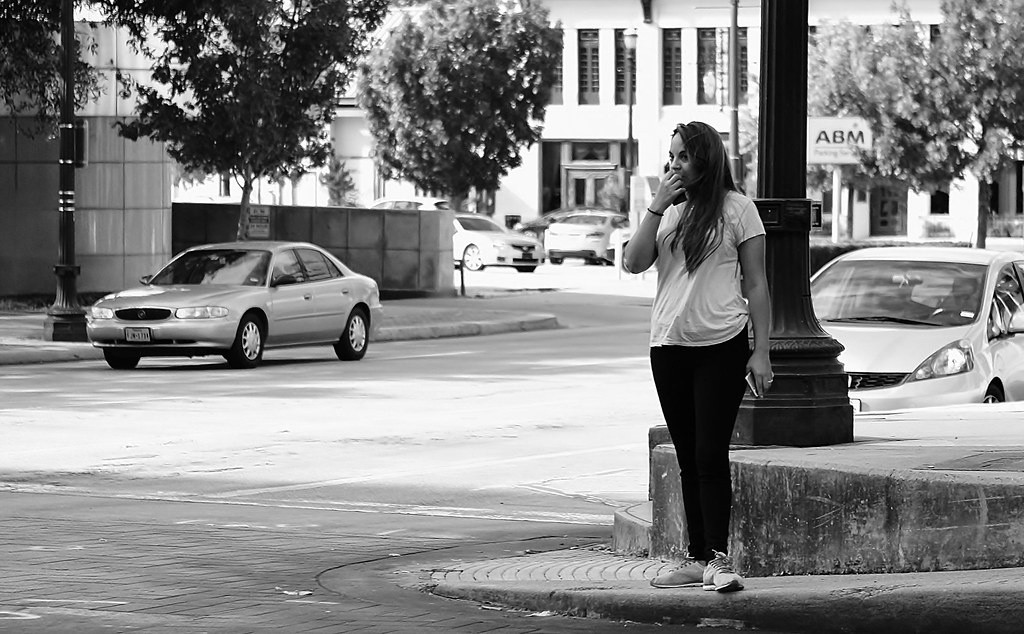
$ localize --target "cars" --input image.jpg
[811,244,1023,411]
[83,240,383,369]
[364,195,631,273]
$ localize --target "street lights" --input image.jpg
[621,27,637,213]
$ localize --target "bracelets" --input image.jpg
[647,208,664,219]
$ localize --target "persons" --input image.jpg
[950,275,994,344]
[623,121,777,593]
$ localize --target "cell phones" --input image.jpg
[745,371,774,396]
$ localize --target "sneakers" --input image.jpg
[702,549,744,591]
[650,557,706,588]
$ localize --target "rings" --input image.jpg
[768,379,774,384]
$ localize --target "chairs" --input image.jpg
[939,279,983,317]
